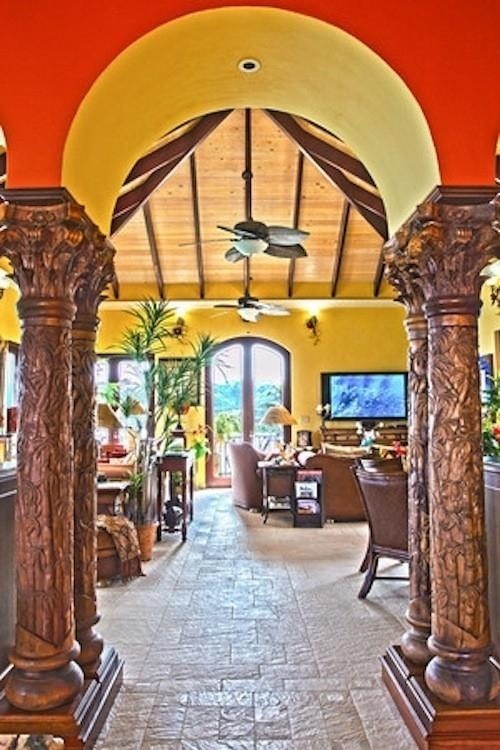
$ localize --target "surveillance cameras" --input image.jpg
[173,327,181,335]
[306,315,318,328]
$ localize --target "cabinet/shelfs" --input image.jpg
[155,445,195,541]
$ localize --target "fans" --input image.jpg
[215,261,291,326]
[178,107,315,262]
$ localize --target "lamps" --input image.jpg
[259,403,298,452]
[237,307,258,323]
[230,239,266,262]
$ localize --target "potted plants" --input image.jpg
[103,295,226,563]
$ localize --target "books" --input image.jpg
[294,468,324,516]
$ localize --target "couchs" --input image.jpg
[301,448,364,522]
[227,444,263,509]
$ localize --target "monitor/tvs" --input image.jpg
[320,371,407,421]
[478,353,492,405]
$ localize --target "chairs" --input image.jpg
[350,463,407,597]
[355,457,405,573]
